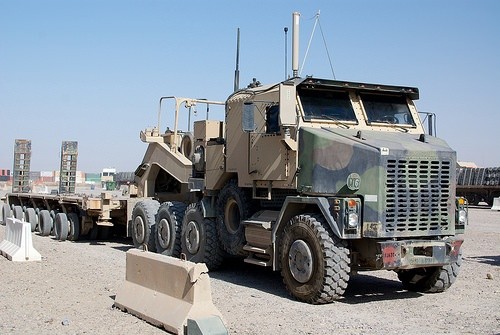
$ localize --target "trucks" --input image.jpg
[456,160,500,208]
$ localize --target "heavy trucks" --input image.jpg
[4,11,471,304]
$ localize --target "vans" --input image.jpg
[100,168,116,187]
